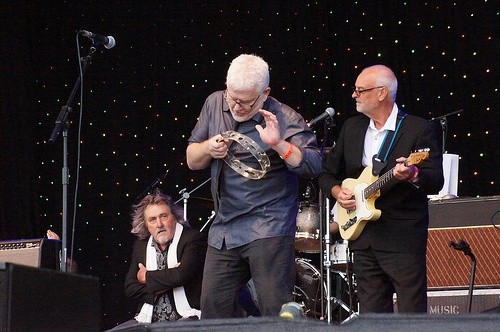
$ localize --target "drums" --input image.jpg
[325,240,350,265]
[296,202,325,253]
[293,256,359,324]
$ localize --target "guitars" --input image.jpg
[337,147,430,240]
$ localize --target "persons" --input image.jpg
[186,54,323,319]
[327,64,444,314]
[124,190,202,323]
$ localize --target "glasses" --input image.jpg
[223,89,265,112]
[352,86,387,96]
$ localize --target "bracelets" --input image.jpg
[280,142,293,160]
[405,163,418,183]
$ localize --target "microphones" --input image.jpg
[80,29,116,49]
[306,107,335,127]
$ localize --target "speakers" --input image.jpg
[0,239,63,271]
[0,262,102,332]
